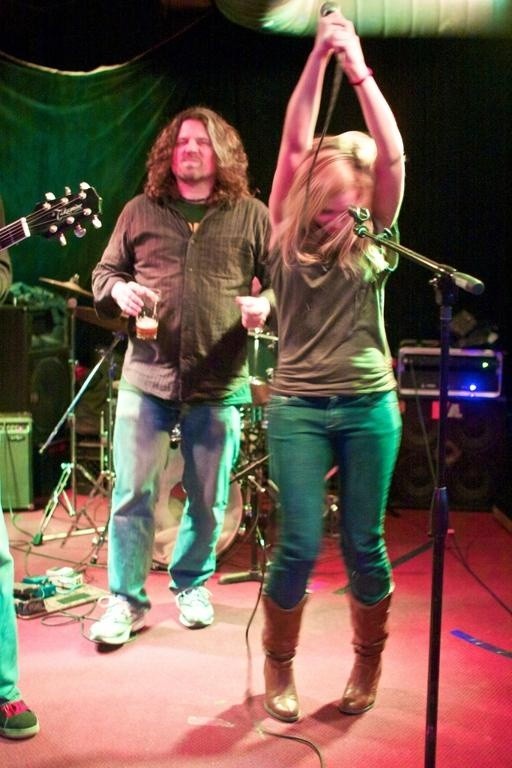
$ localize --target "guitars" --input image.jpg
[0,180,104,251]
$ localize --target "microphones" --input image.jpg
[320,1,346,62]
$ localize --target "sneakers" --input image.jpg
[89,594,150,648]
[170,583,215,628]
[2,700,40,741]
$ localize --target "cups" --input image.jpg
[243,297,266,336]
[136,287,160,340]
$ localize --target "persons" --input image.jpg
[0,506,43,740]
[88,105,277,649]
[259,9,407,723]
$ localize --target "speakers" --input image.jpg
[0,411,35,510]
[396,395,506,513]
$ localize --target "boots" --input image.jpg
[262,589,306,725]
[337,584,395,713]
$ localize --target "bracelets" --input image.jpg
[347,66,375,87]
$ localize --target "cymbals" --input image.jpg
[39,277,93,299]
[66,305,128,332]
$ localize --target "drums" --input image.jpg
[151,427,244,569]
[248,333,279,405]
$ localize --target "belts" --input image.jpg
[299,393,366,407]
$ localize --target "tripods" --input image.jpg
[218,475,313,594]
[31,295,108,545]
[38,334,124,548]
